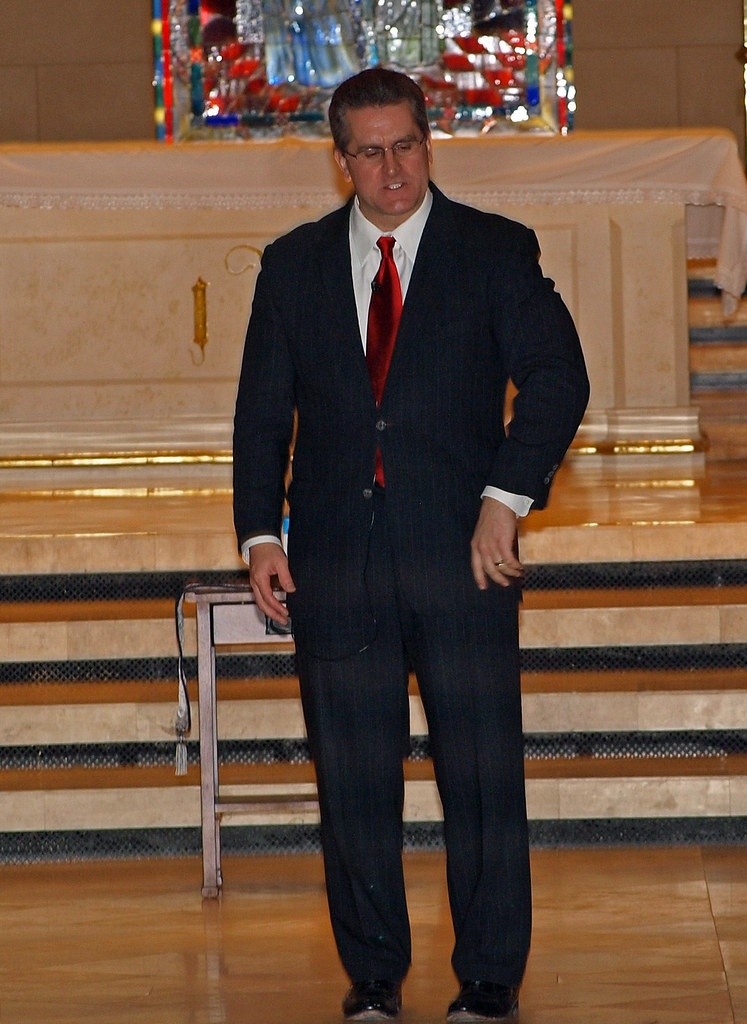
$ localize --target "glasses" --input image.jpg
[343,131,427,167]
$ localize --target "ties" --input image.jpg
[366,235,403,487]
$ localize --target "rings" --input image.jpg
[496,560,504,566]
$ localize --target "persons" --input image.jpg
[233,69,591,1024]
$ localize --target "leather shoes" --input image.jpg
[342,982,402,1021]
[446,974,520,1024]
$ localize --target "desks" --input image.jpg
[185,585,319,900]
[0,128,747,468]
